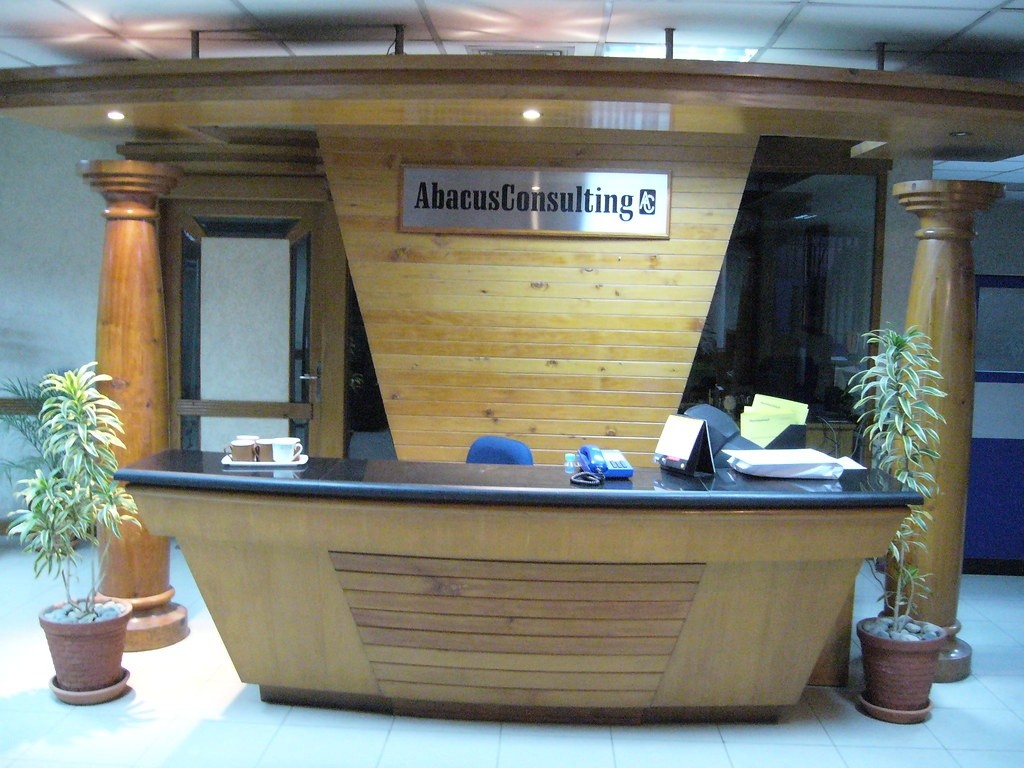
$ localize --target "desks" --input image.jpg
[805,417,857,458]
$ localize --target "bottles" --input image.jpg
[564,453,577,466]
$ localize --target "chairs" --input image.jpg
[467,435,534,466]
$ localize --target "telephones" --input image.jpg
[577,444,634,478]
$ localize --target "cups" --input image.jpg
[224,440,255,461]
[236,435,259,440]
[272,438,303,461]
[253,439,275,462]
[293,438,300,461]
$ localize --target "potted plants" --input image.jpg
[1,363,144,705]
[847,322,945,724]
